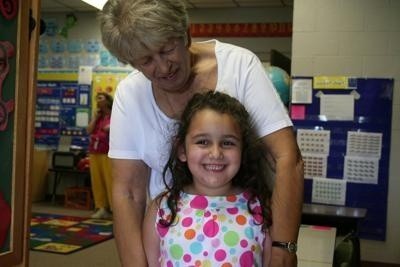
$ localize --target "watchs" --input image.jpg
[271,240,297,256]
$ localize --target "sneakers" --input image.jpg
[91,209,111,219]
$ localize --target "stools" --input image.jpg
[64,185,91,210]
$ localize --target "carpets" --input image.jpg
[28,212,114,253]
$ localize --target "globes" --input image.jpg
[265,65,290,105]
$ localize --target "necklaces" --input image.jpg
[165,92,177,116]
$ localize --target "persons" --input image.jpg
[98,0,305,266]
[84,92,114,219]
[141,86,276,266]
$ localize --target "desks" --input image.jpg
[47,167,91,204]
[300,199,370,267]
